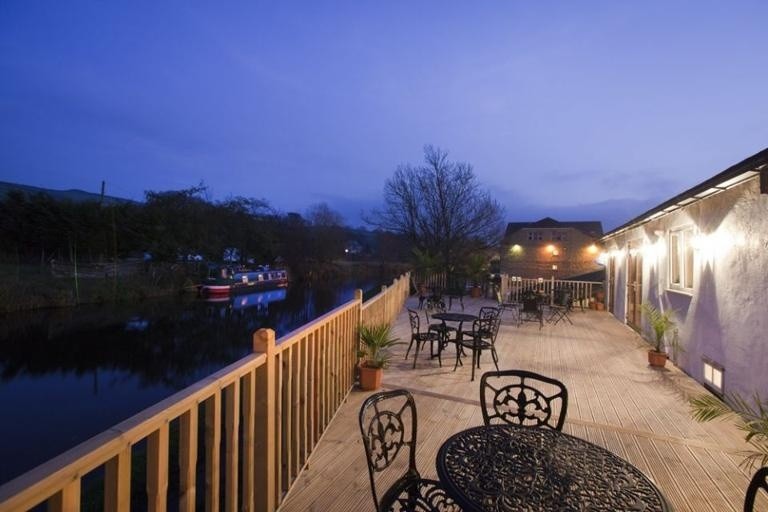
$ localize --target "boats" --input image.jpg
[202,286,289,318]
[200,263,289,294]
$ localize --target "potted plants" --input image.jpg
[466,254,486,297]
[638,303,685,366]
[351,321,407,391]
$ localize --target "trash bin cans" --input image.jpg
[561,289,570,305]
[486,282,495,298]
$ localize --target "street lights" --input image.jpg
[550,263,558,306]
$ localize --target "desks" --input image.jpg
[435,423,675,512]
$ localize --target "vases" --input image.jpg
[596,303,605,311]
[596,290,606,299]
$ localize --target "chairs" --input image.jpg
[479,369,568,433]
[358,388,465,511]
[405,282,577,380]
